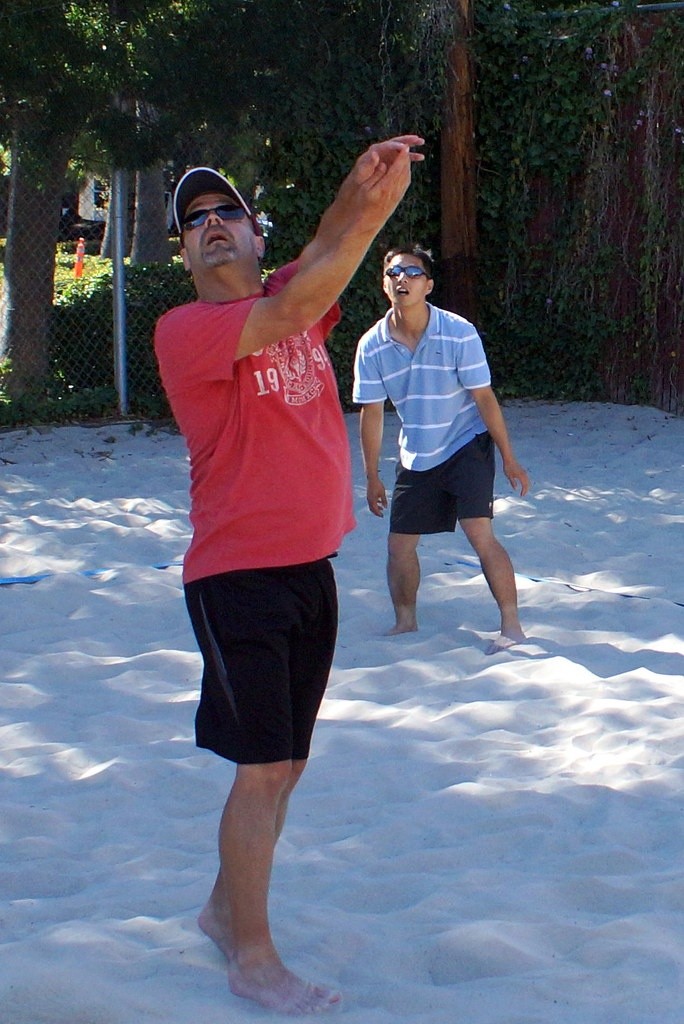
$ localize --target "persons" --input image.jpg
[153,134,425,1014]
[351,245,527,654]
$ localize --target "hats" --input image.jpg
[173,167,262,236]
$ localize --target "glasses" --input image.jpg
[384,265,431,280]
[180,204,246,248]
